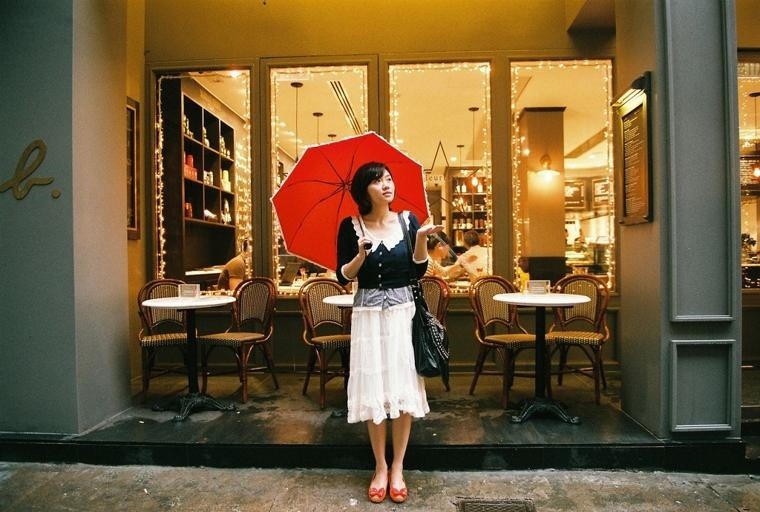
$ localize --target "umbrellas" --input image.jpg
[269,130,430,273]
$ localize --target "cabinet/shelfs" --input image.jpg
[161,79,236,270]
[126,96,141,240]
[446,165,492,255]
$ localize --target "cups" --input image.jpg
[467,206,471,212]
[453,223,472,230]
[186,155,194,167]
[222,170,229,181]
[185,203,192,217]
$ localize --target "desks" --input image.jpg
[140,296,240,420]
[320,294,353,310]
[495,291,587,427]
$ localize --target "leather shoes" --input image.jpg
[369,468,409,504]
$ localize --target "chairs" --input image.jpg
[543,274,610,405]
[417,277,453,392]
[195,276,280,406]
[298,278,352,409]
[136,278,200,398]
[469,273,537,410]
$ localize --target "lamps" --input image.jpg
[535,154,561,181]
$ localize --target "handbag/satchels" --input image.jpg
[410,299,453,376]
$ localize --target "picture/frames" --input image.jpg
[608,69,653,227]
[749,91,760,179]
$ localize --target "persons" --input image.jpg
[565,234,587,261]
[423,229,478,282]
[332,159,446,503]
[216,239,254,291]
[454,229,493,277]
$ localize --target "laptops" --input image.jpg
[276,262,300,285]
